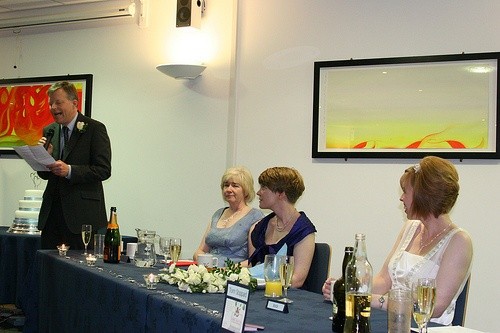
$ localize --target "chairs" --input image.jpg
[298,243,330,294]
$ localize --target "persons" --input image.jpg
[323,156,473,327]
[193,167,265,270]
[247,167,318,289]
[37,82,112,250]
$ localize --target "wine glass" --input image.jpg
[410,277,436,333]
[159,237,171,272]
[169,238,181,265]
[278,256,295,304]
[81,225,92,255]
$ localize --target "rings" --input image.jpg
[55,170,56,172]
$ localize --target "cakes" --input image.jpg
[15,189,43,225]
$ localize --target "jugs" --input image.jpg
[133,228,161,268]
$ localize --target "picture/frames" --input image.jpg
[0,74,93,157]
[312,52,500,161]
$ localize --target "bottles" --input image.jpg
[344,233,373,333]
[103,206,121,264]
[332,246,361,333]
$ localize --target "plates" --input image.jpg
[257,278,266,289]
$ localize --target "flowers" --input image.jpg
[158,257,257,294]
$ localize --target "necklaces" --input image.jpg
[276,208,296,232]
[223,211,239,224]
[419,221,451,253]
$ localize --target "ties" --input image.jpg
[61,126,69,160]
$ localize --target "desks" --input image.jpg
[36,249,484,333]
[0,226,138,302]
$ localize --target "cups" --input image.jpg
[86,254,97,266]
[127,243,137,259]
[57,246,69,256]
[264,254,283,300]
[386,287,413,333]
[94,234,105,259]
[143,274,160,290]
[198,254,218,268]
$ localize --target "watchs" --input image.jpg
[378,295,385,310]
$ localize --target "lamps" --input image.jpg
[154,64,207,79]
[0,3,135,31]
